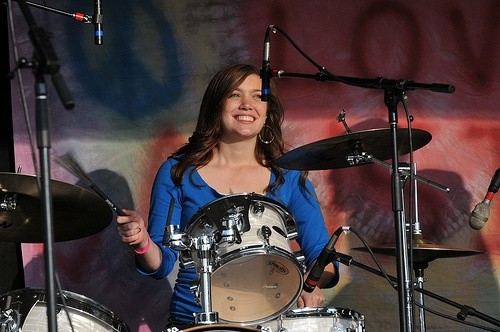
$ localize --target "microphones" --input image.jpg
[261,31,270,103]
[303,227,344,294]
[94,0,104,46]
[469,168,500,230]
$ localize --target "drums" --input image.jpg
[251,306,366,332]
[0,288,136,332]
[185,190,307,324]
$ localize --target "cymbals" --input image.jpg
[0,172,113,243]
[350,235,487,264]
[273,127,433,170]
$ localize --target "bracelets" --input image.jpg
[134,234,152,256]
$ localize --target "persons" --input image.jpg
[117,62,340,332]
